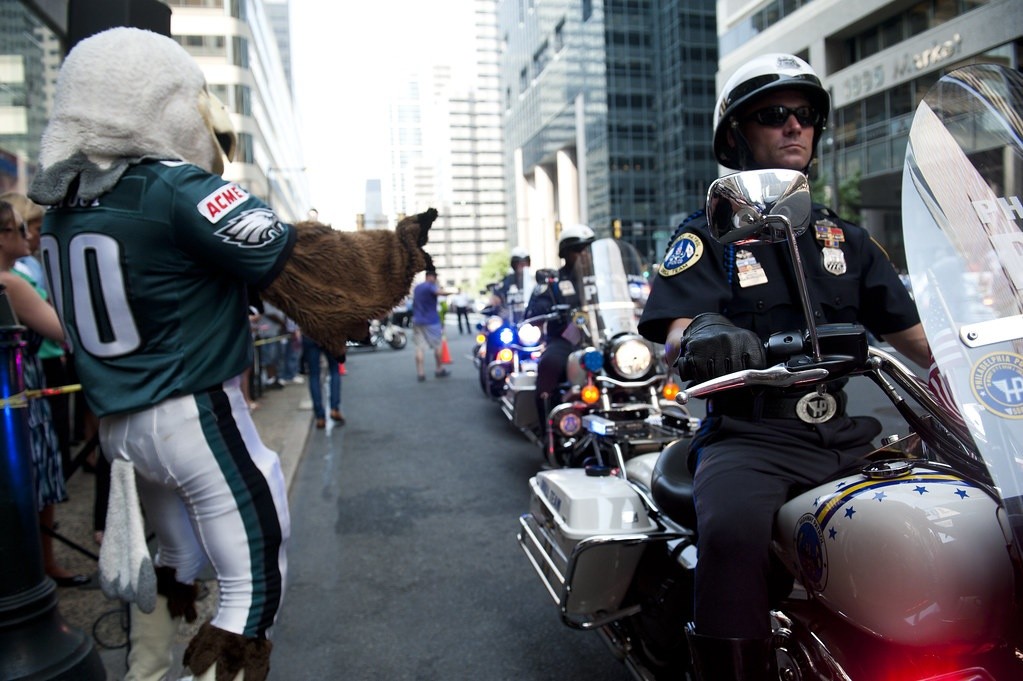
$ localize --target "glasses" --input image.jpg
[573,244,584,252]
[0,222,28,236]
[736,106,820,127]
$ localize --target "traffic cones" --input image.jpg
[439,335,458,367]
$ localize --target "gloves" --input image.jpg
[673,313,766,385]
[550,305,572,320]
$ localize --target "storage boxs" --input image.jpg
[504,378,539,427]
[522,468,656,613]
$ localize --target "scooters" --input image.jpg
[346,312,409,351]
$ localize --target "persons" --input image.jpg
[492,224,648,470]
[413,271,453,381]
[451,288,475,333]
[711,192,735,236]
[391,298,413,327]
[639,54,932,681]
[30,26,439,681]
[0,192,98,586]
[300,335,345,429]
[240,302,307,412]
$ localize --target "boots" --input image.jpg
[684,622,779,681]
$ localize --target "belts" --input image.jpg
[712,394,846,423]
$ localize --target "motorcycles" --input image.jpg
[470,237,701,471]
[509,65,1019,681]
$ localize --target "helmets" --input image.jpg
[510,247,531,270]
[559,225,595,257]
[712,53,830,169]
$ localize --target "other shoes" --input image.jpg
[54,573,92,587]
[317,418,325,427]
[293,376,305,384]
[331,409,343,421]
[338,364,345,374]
[417,375,425,381]
[436,369,450,376]
